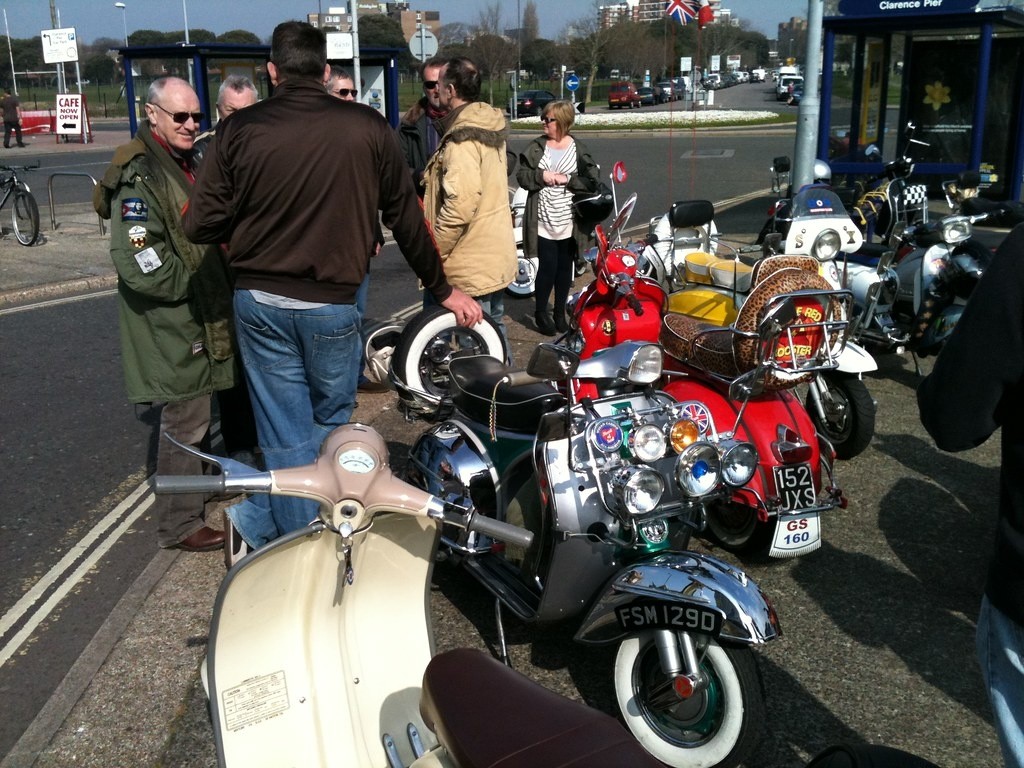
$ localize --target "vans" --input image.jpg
[608,82,641,108]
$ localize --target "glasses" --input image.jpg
[542,115,555,123]
[331,88,357,97]
[424,81,438,89]
[153,103,204,124]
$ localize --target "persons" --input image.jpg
[516,100,598,336]
[395,56,447,199]
[420,57,519,366]
[917,224,1023,767]
[182,19,483,571]
[0,88,24,148]
[94,77,242,551]
[785,81,794,108]
[184,74,259,173]
[325,65,389,408]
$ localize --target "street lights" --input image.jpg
[114,2,129,48]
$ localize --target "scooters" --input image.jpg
[149,425,680,768]
[501,120,992,564]
[358,309,781,768]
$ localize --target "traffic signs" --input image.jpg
[54,95,82,134]
[39,27,80,63]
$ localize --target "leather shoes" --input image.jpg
[175,525,225,550]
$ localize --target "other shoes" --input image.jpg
[4,144,10,148]
[18,142,24,147]
[553,312,568,333]
[535,311,557,336]
[356,380,389,393]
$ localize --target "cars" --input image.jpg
[506,90,556,117]
[636,68,768,107]
[770,66,804,102]
[792,82,804,105]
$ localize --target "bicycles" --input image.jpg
[0,159,41,247]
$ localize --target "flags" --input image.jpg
[665,0,720,27]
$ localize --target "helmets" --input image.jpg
[572,182,614,223]
[814,159,832,183]
